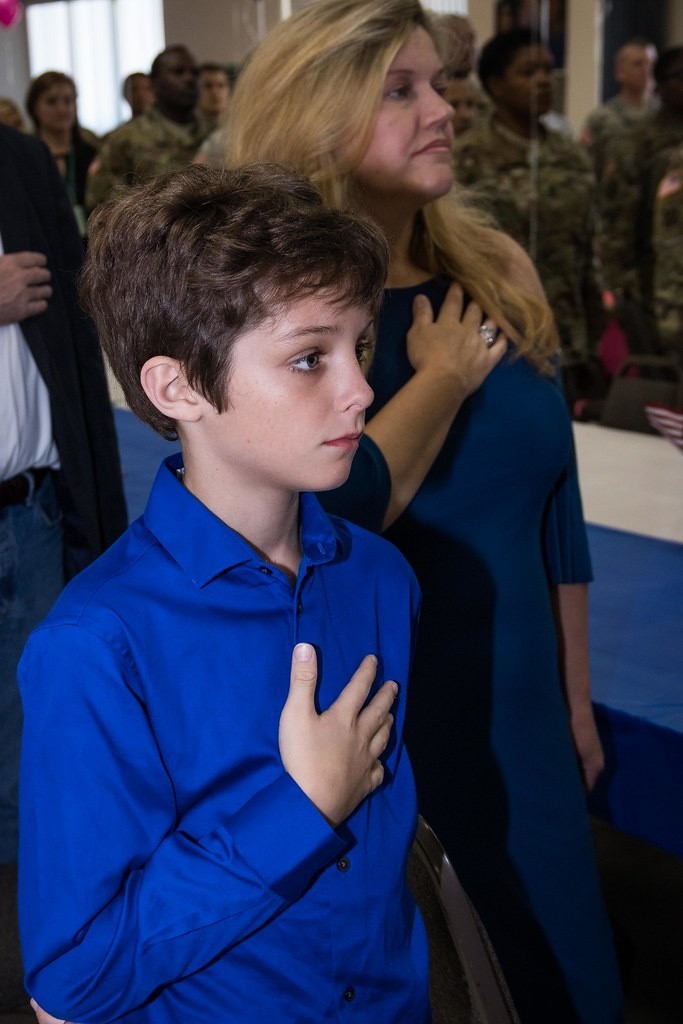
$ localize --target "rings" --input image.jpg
[480,326,494,346]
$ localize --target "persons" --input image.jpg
[632,46,683,384]
[85,47,216,220]
[197,65,230,131]
[225,0,625,1024]
[0,98,26,133]
[432,15,485,123]
[17,165,431,1024]
[0,127,130,1024]
[123,72,154,122]
[27,72,100,239]
[579,36,659,335]
[451,26,617,420]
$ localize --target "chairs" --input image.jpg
[600,355,683,441]
